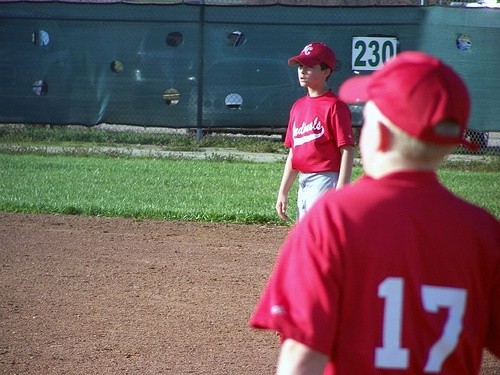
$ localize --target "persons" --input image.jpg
[248,52,500,375]
[274,41,353,228]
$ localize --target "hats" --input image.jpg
[338,50,480,152]
[288,41,336,71]
